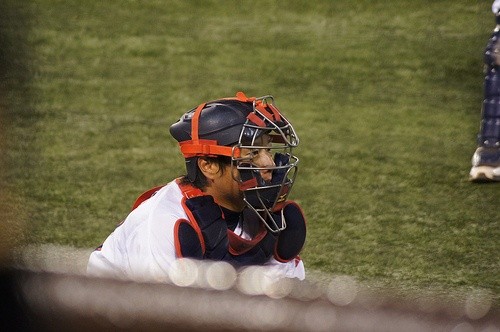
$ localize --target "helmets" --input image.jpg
[169,90,299,183]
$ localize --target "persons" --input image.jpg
[87,92,305,283]
[467,0,500,183]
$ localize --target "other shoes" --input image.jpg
[468,147,500,183]
